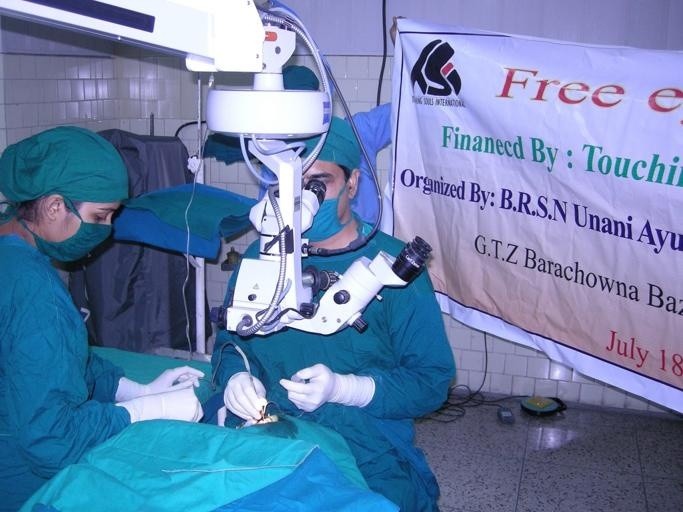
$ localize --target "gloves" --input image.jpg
[224,371,266,422]
[279,363,376,413]
[116,365,204,399]
[115,387,205,425]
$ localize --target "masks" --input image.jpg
[29,198,114,263]
[303,182,354,243]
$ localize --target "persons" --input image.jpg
[210,118,456,512]
[0,126,202,512]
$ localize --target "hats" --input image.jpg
[301,117,362,171]
[0,126,130,204]
